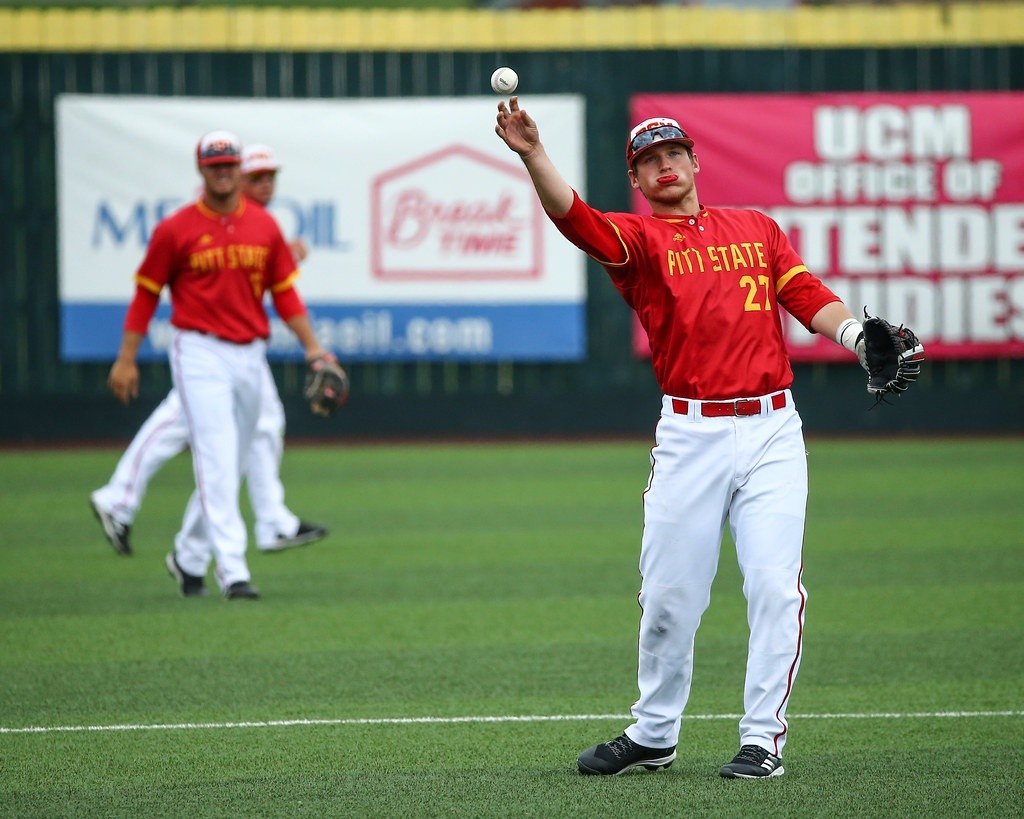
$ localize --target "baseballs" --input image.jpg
[490,66,519,94]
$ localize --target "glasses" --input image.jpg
[202,145,239,158]
[629,126,688,151]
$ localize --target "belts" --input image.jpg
[672,392,785,417]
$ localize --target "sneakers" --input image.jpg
[720,744,785,779]
[577,734,677,776]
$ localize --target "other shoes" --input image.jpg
[265,521,326,551]
[89,494,130,556]
[223,583,259,599]
[165,552,207,599]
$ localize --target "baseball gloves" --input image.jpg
[861,304,926,412]
[302,351,351,418]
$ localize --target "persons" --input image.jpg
[496,96,924,778]
[90,142,328,554]
[107,129,347,599]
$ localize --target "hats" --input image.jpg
[626,116,695,170]
[240,145,277,174]
[196,130,241,166]
